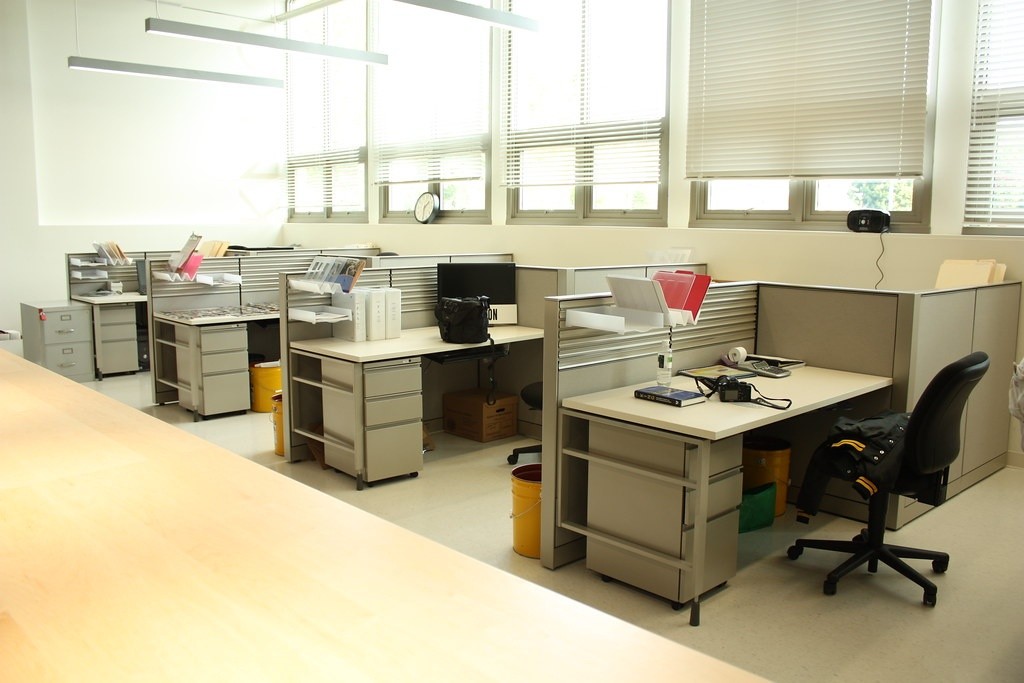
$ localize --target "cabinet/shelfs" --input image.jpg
[19,298,96,383]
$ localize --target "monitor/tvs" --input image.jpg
[438,264,516,305]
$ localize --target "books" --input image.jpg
[328,257,366,293]
[634,353,806,409]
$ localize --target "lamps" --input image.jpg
[145,0,388,68]
[67,0,284,91]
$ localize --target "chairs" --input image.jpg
[786,350,990,608]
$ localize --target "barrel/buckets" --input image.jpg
[743,442,790,516]
[510,463,542,559]
[272,394,284,455]
[249,361,282,413]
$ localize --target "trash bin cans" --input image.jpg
[269,394,283,456]
[511,465,543,560]
[250,361,281,413]
[742,441,790,518]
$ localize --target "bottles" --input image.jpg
[658,340,672,386]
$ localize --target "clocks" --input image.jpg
[413,192,439,224]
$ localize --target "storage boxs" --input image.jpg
[443,388,519,442]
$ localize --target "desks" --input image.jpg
[153,305,280,423]
[561,366,892,627]
[70,292,147,381]
[290,325,544,491]
[1,348,772,683]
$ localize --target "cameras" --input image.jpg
[718,382,751,403]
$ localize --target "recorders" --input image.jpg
[846,209,890,233]
